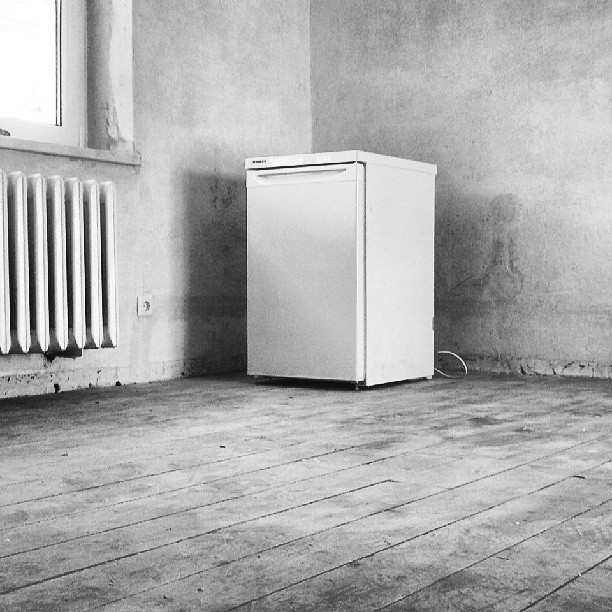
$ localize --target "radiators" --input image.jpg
[2,168,124,358]
